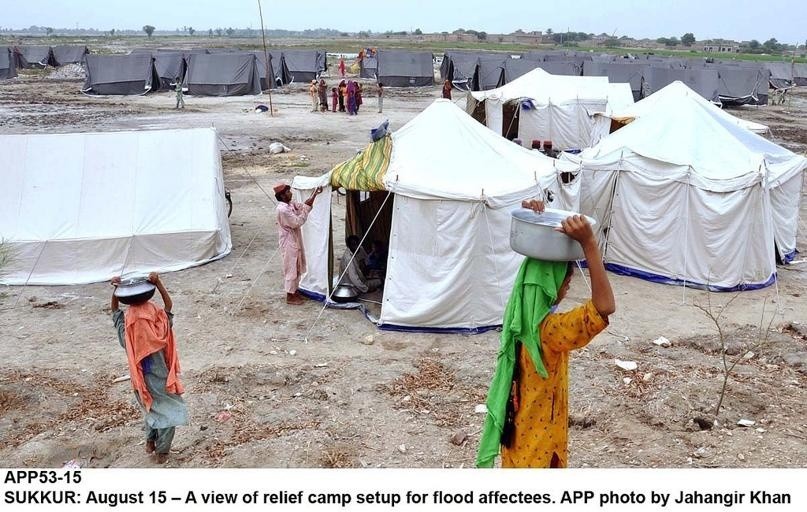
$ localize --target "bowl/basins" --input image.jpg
[510,209,596,263]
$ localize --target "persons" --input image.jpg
[336,235,383,294]
[308,78,384,115]
[271,179,324,308]
[169,77,184,110]
[108,272,184,464]
[498,204,616,468]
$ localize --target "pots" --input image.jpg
[113,277,156,304]
[332,283,360,303]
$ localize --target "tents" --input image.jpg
[290,97,580,336]
[437,46,807,295]
[0,126,232,287]
[0,43,436,96]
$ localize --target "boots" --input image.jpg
[294,289,307,301]
[286,292,303,304]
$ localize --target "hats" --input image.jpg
[513,137,522,144]
[272,182,285,193]
[544,140,552,146]
[532,139,540,145]
